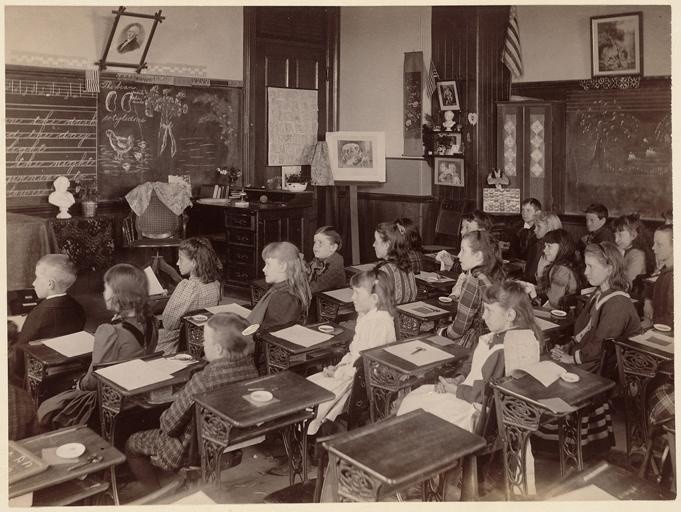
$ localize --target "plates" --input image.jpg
[59,443,85,460]
[251,390,272,403]
[317,323,335,334]
[196,197,231,206]
[190,312,209,321]
[561,373,581,384]
[548,309,568,317]
[438,295,454,304]
[653,321,671,333]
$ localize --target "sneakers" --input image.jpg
[269,462,295,476]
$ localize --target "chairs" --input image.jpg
[39,217,116,289]
[124,182,191,286]
[417,199,469,252]
[262,414,394,504]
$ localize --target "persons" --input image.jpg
[118,25,139,53]
[442,110,457,130]
[49,176,76,220]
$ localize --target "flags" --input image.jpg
[500,8,526,81]
[425,60,442,101]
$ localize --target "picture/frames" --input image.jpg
[433,157,466,188]
[322,129,390,185]
[431,131,466,158]
[94,6,168,76]
[436,79,463,111]
[589,11,646,79]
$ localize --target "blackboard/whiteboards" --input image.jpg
[7,439,48,485]
[5,64,246,213]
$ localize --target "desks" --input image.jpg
[93,350,209,445]
[491,358,615,505]
[251,278,271,305]
[194,185,316,295]
[180,303,255,360]
[419,253,461,270]
[360,331,469,422]
[259,320,356,377]
[611,322,674,479]
[191,369,336,496]
[413,270,461,297]
[7,312,32,342]
[524,282,592,358]
[321,408,487,504]
[19,330,96,413]
[498,239,526,278]
[147,293,171,351]
[317,286,356,320]
[7,424,126,504]
[344,260,383,284]
[391,296,459,336]
[627,272,661,319]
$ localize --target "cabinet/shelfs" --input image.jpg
[494,98,567,215]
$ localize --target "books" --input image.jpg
[214,183,231,199]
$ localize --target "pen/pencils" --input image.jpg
[307,320,330,328]
[67,460,91,471]
[411,348,422,355]
[424,306,435,312]
[248,387,281,391]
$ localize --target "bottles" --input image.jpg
[263,178,278,189]
